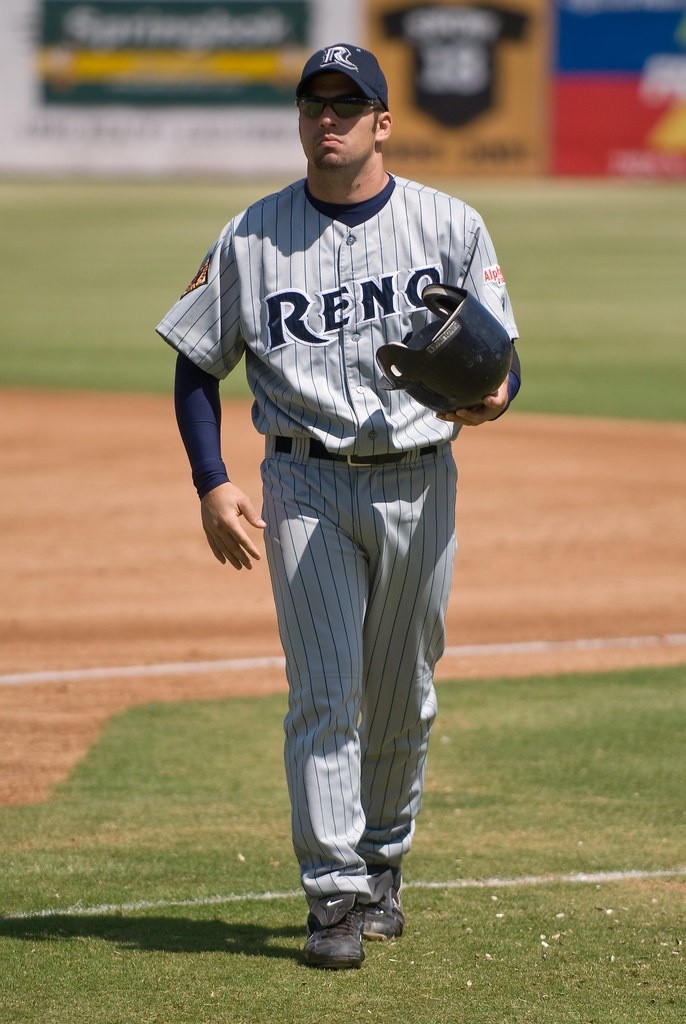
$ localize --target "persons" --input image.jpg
[154,44,521,973]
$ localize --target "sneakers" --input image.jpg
[364,862,405,943]
[302,893,365,970]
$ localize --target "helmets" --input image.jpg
[376,284,514,413]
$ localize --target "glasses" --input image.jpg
[295,93,384,119]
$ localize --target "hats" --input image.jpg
[294,44,390,114]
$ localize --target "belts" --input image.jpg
[275,436,437,467]
